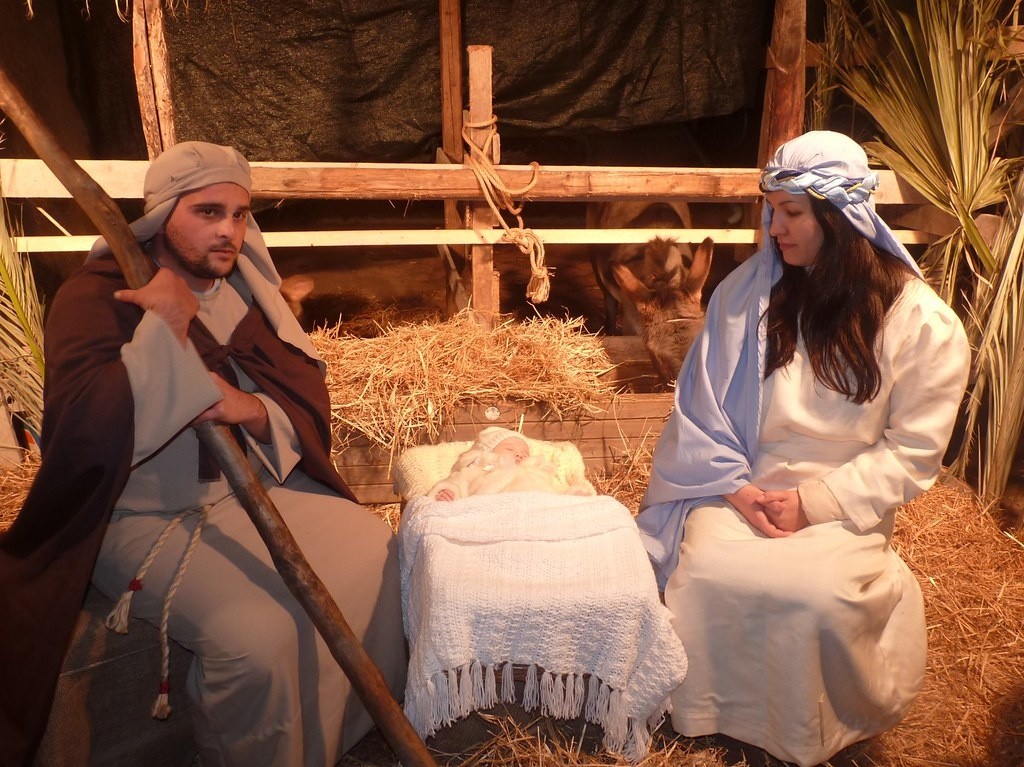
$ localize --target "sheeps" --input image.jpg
[588,200,714,388]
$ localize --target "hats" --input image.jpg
[470,427,530,459]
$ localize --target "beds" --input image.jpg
[391,440,598,679]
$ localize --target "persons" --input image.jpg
[428,425,588,502]
[0,142,405,767]
[640,129,972,767]
